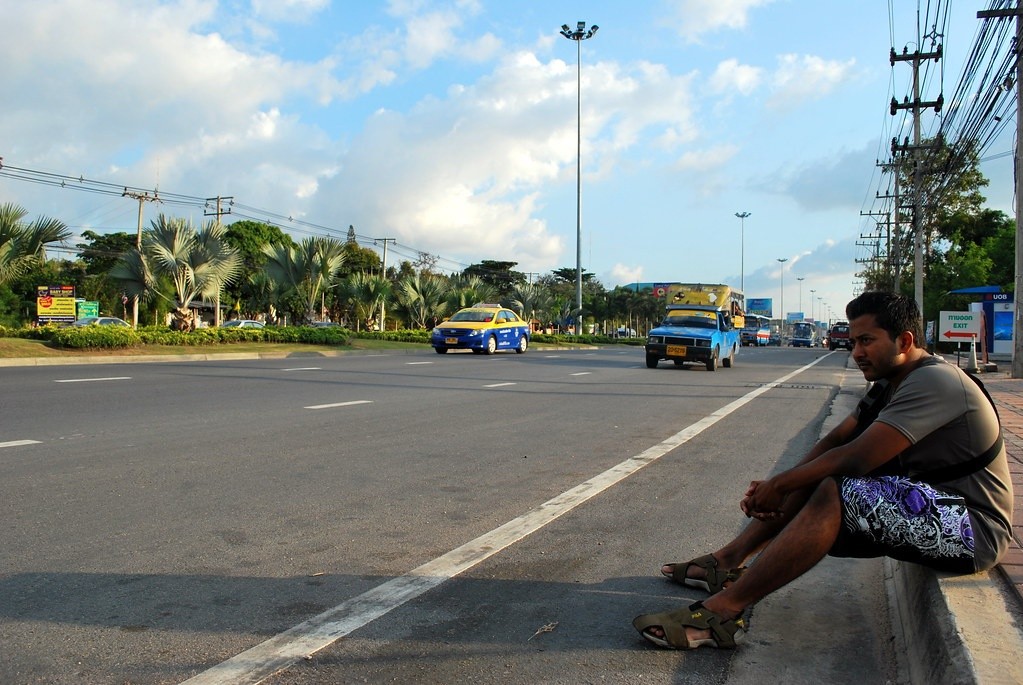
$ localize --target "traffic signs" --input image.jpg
[940,311,980,342]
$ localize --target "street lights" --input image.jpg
[561,19,599,335]
[736,211,751,292]
[797,277,805,313]
[776,258,788,331]
[810,289,842,324]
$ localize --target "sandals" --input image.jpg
[632,600,746,649]
[660,552,739,595]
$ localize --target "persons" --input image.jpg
[628,286,1015,651]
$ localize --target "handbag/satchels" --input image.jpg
[841,414,909,478]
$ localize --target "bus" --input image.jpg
[739,314,771,348]
[828,322,853,351]
[792,322,816,349]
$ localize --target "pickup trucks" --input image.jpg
[645,306,741,371]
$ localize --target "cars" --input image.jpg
[432,303,530,355]
[223,320,264,330]
[767,334,782,347]
[306,323,344,329]
[71,316,132,329]
